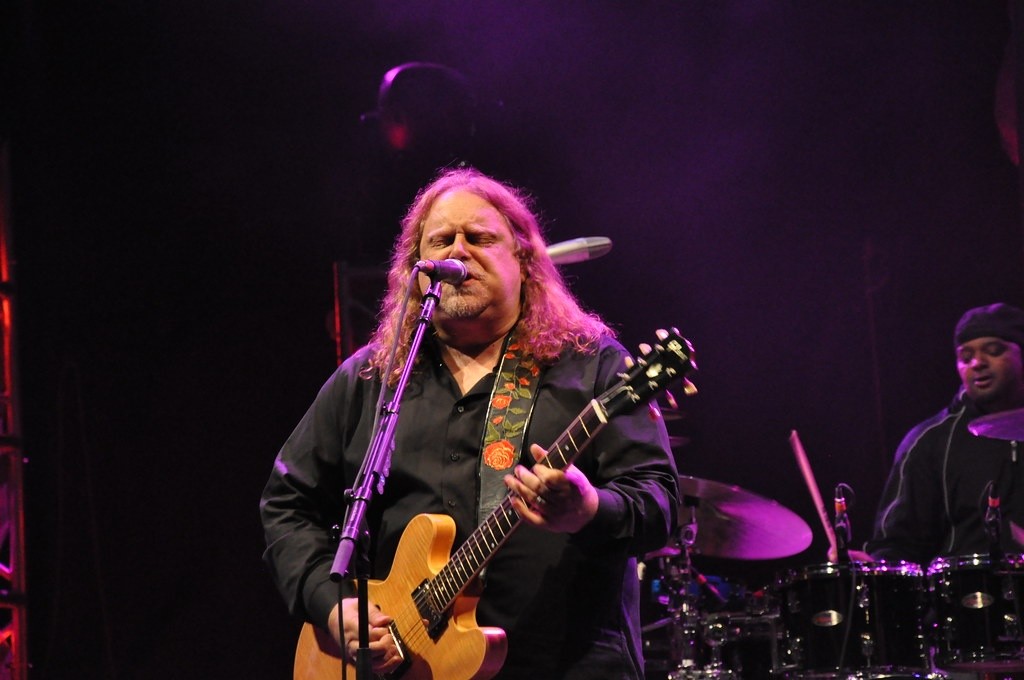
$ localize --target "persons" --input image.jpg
[866,305,1024,565]
[257,167,680,680]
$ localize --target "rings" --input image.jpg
[530,496,542,506]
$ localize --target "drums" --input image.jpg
[664,611,777,680]
[922,551,1024,680]
[770,558,922,678]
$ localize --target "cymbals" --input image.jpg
[967,408,1024,442]
[676,473,815,559]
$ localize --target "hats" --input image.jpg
[954,303,1024,350]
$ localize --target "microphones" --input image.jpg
[985,482,1001,526]
[414,258,468,287]
[834,485,853,543]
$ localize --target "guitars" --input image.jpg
[288,328,700,680]
